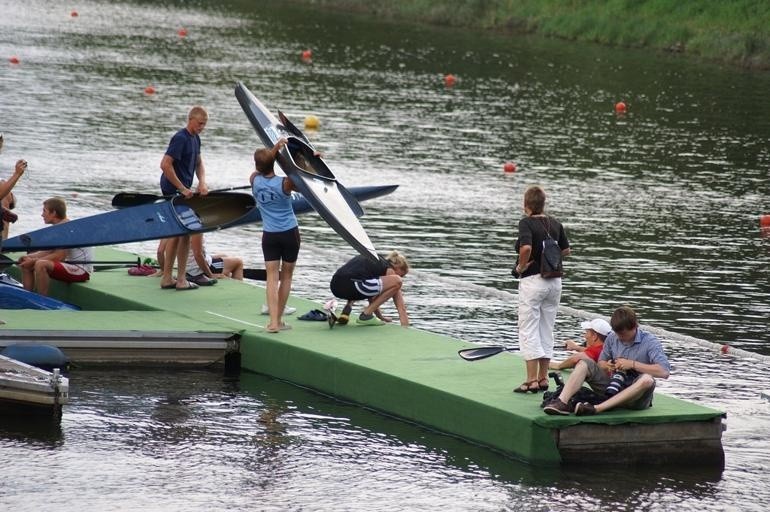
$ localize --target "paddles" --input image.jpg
[113,185,252,209]
[94,264,282,280]
[459,340,586,361]
[277,109,364,218]
[0,254,140,273]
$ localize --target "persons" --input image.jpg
[0,135,28,244]
[146,231,244,282]
[17,197,94,296]
[249,138,323,333]
[511,184,571,393]
[547,317,614,376]
[330,249,412,328]
[158,107,209,291]
[543,305,671,416]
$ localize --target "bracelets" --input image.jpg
[632,359,637,370]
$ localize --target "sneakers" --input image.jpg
[261,303,297,315]
[543,398,571,415]
[185,272,218,286]
[574,402,596,416]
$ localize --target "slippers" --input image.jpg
[265,322,280,334]
[159,277,177,289]
[280,321,293,331]
[356,314,386,326]
[298,308,327,321]
[337,314,349,325]
[176,281,200,293]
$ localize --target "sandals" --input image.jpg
[538,377,549,391]
[513,379,539,393]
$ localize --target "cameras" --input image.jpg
[605,360,639,397]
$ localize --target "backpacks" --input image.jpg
[530,215,563,279]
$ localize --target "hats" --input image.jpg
[580,318,612,337]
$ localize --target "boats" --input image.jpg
[0,352,71,420]
[235,80,382,269]
[0,184,398,252]
[0,272,83,317]
